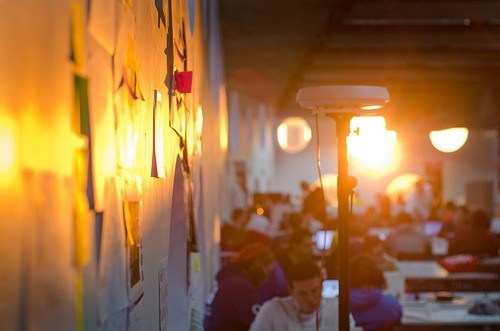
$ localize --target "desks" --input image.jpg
[221,208,499,326]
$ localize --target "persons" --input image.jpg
[349,288,403,331]
[250,261,356,331]
[224,180,498,294]
[204,242,276,331]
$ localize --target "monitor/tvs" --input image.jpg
[315,229,337,251]
